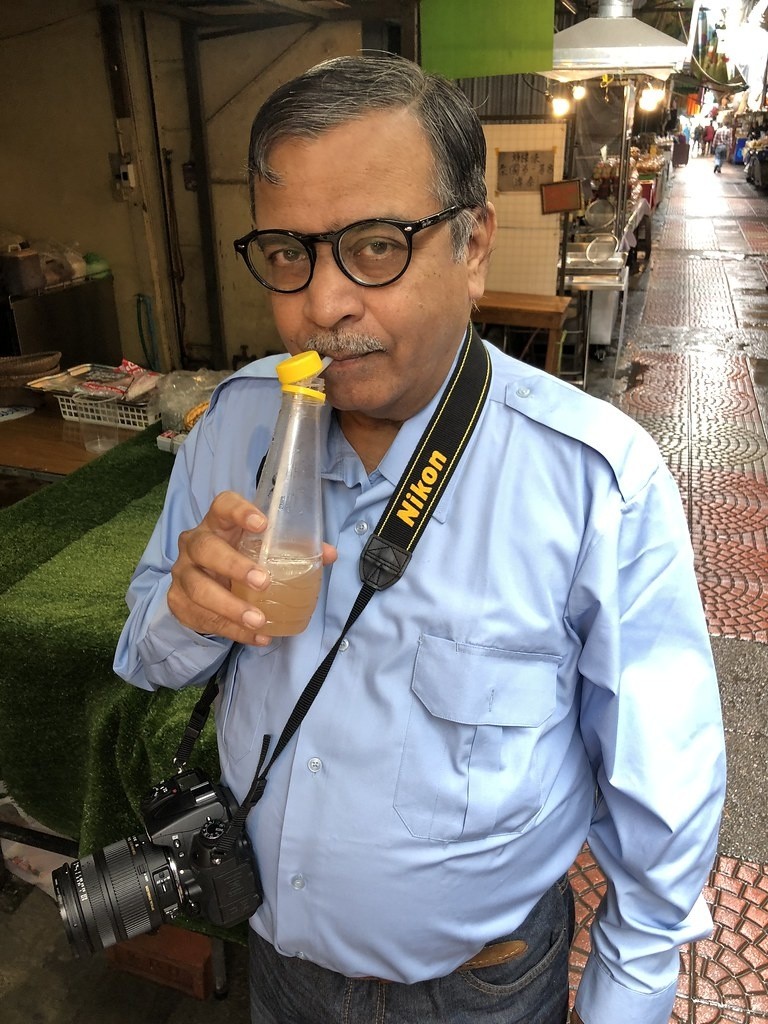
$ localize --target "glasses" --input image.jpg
[232,202,465,294]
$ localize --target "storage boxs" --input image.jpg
[59,396,161,434]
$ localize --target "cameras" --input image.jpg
[51,767,265,959]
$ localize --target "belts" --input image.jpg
[460,940,528,970]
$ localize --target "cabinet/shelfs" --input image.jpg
[0,269,124,367]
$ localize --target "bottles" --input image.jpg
[232,352,330,633]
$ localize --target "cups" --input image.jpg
[73,390,119,454]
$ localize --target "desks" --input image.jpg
[470,291,571,376]
[0,405,231,1001]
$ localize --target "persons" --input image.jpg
[114,50,725,1024]
[677,120,768,173]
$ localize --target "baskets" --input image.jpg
[52,393,165,431]
